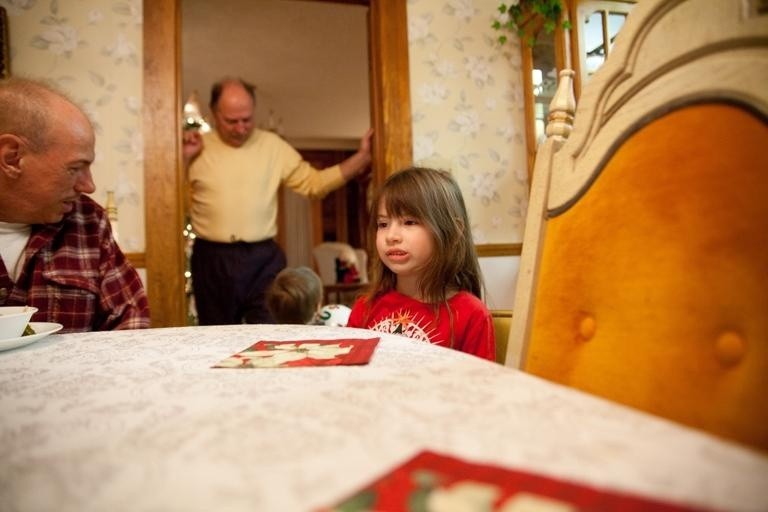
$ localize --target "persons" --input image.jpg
[182,77,376,326]
[342,164,497,364]
[0,75,157,332]
[266,267,327,324]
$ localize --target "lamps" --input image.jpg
[498,1,766,458]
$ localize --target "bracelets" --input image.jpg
[0,306,40,337]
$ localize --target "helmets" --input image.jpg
[0,320,64,352]
